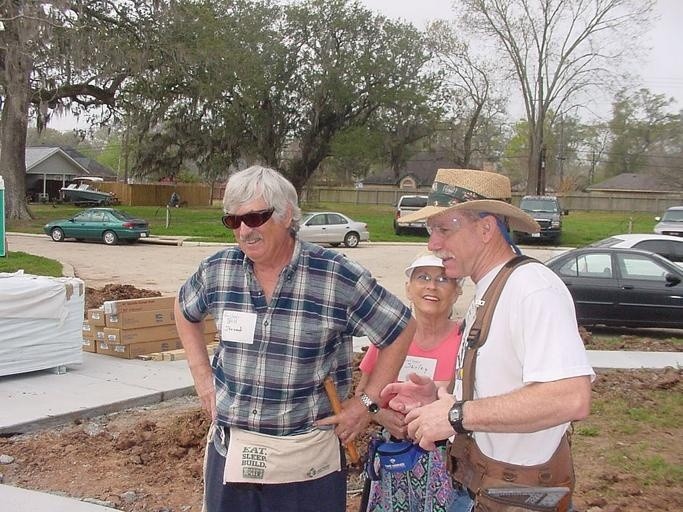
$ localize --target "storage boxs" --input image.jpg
[82,297,217,360]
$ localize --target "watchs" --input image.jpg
[448,400,473,434]
[360,392,379,414]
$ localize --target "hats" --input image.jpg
[396,168,541,233]
[404,250,463,278]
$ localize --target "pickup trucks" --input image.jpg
[393,195,429,234]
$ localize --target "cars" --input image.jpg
[543,206,683,328]
[514,196,568,245]
[44,207,150,245]
[296,212,369,248]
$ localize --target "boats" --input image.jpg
[60,177,113,201]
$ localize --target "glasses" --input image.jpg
[222,207,276,229]
[416,272,452,284]
[423,217,477,234]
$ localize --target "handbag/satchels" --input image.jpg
[359,439,474,512]
[223,427,342,484]
[446,433,575,511]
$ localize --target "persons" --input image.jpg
[395,167,597,512]
[173,165,417,512]
[356,249,477,512]
